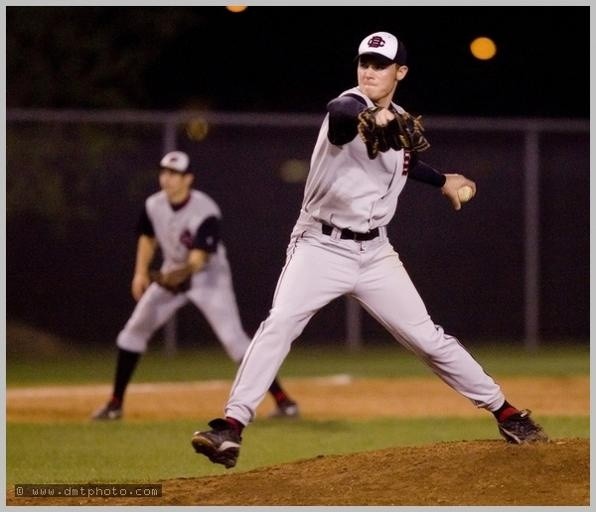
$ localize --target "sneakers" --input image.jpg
[498,409,547,444]
[97,405,122,419]
[192,419,242,468]
[278,396,297,415]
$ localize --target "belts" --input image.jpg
[322,224,379,241]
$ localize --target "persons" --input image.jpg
[191,30,548,468]
[90,150,299,418]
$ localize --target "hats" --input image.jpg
[158,151,191,175]
[354,32,406,65]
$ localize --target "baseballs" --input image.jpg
[458,186,473,202]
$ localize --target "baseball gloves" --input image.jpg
[153,265,194,294]
[358,107,431,159]
